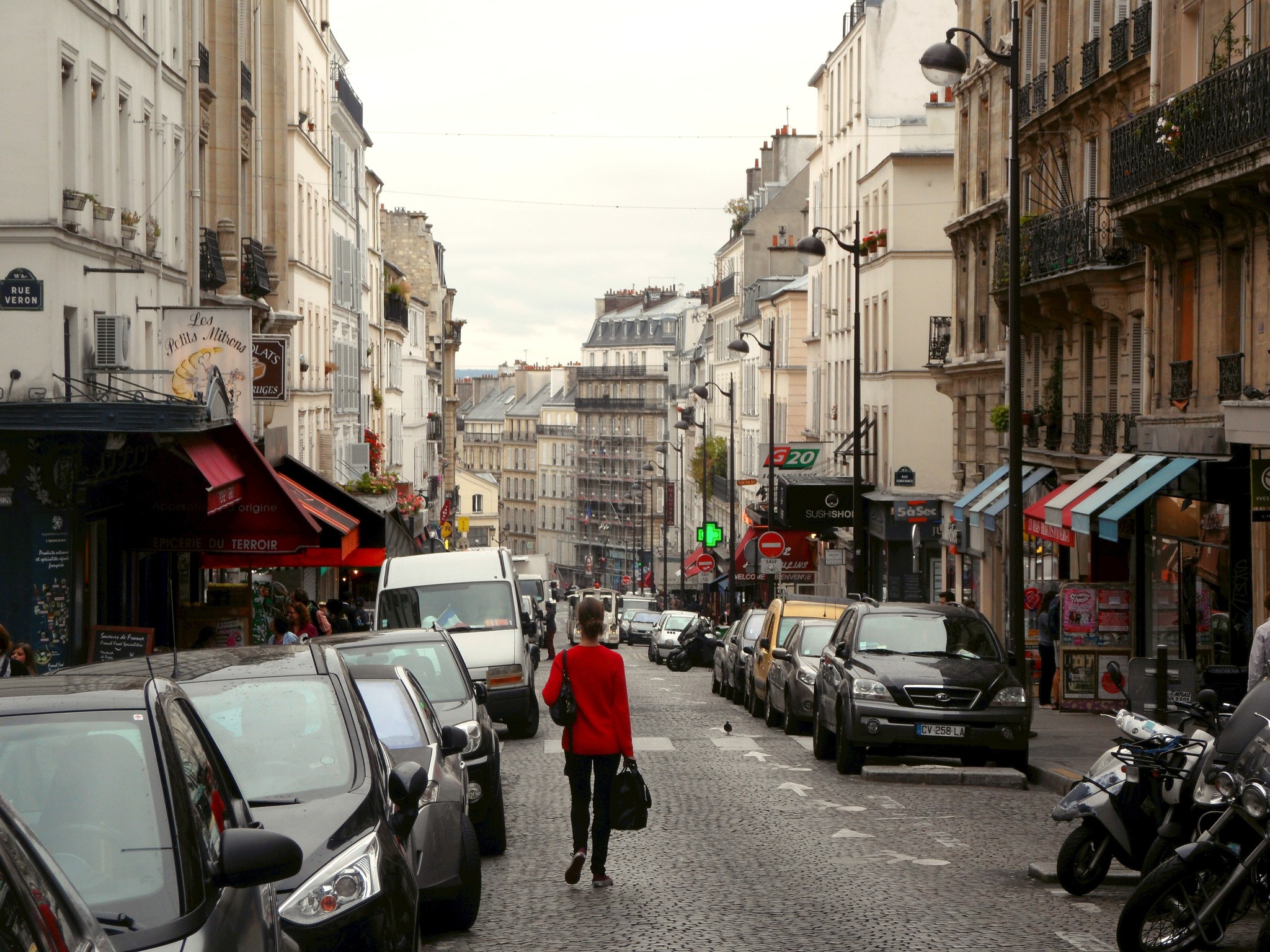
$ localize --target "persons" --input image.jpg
[541,600,635,891]
[1038,593,1057,709]
[268,586,368,647]
[659,593,763,626]
[541,602,557,661]
[0,624,28,678]
[10,642,39,675]
[1049,579,1072,708]
[1246,594,1269,692]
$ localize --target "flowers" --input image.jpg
[364,427,385,473]
[396,492,424,516]
[876,229,887,240]
[371,475,396,490]
[1154,96,1182,152]
[862,230,877,245]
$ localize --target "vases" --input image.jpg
[867,244,878,253]
[952,470,964,480]
[94,204,115,220]
[1022,414,1032,426]
[971,471,983,483]
[878,237,887,247]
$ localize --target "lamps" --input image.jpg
[756,485,778,502]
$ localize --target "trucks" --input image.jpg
[508,553,560,621]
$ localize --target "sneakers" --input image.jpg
[592,873,613,887]
[565,848,586,884]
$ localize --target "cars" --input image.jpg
[1,621,512,952]
[620,593,1032,779]
[521,595,546,648]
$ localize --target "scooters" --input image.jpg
[665,611,727,672]
[1050,651,1270,951]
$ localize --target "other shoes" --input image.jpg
[544,657,551,660]
[1052,702,1059,710]
[1039,704,1052,709]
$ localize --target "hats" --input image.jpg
[355,597,364,602]
[318,602,326,606]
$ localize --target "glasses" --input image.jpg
[288,611,297,614]
[270,622,274,624]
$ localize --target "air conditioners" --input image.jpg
[94,313,132,369]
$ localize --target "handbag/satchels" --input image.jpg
[718,621,728,626]
[612,757,652,831]
[549,676,578,727]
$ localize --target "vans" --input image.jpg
[374,543,541,739]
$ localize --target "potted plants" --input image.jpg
[120,207,141,240]
[66,190,104,213]
[1039,405,1054,426]
[145,215,161,248]
[859,243,868,256]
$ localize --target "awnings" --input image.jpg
[952,462,1009,523]
[173,434,242,514]
[969,466,1033,528]
[683,567,700,577]
[1024,481,1100,548]
[1071,455,1167,536]
[1043,453,1136,529]
[983,466,1054,532]
[271,471,360,562]
[1099,452,1197,543]
[684,546,710,572]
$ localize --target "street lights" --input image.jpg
[727,320,777,603]
[918,1,1026,689]
[601,372,738,625]
[794,204,867,600]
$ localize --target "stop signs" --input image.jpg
[696,553,715,573]
[622,576,631,585]
[758,531,785,559]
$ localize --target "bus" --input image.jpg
[564,580,623,649]
